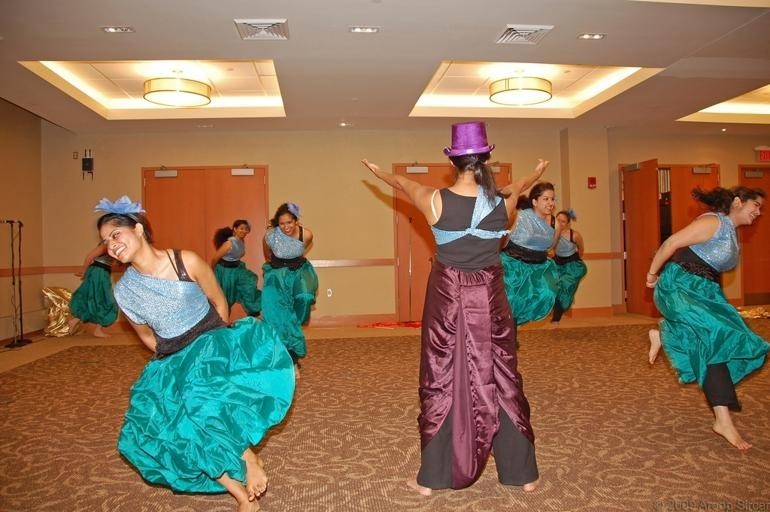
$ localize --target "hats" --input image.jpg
[442,122,495,158]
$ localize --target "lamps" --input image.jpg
[142,77,212,108]
[489,76,554,108]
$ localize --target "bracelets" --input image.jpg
[648,270,658,276]
[646,280,658,285]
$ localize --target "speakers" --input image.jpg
[82,157,93,170]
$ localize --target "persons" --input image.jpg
[642,181,769,451]
[498,178,560,331]
[359,145,549,499]
[261,203,318,382]
[92,207,295,512]
[206,219,263,322]
[64,239,124,339]
[550,205,588,327]
